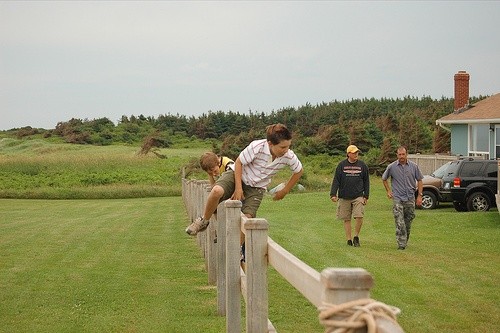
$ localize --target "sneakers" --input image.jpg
[348,240,353,246]
[185,215,210,236]
[397,246,406,250]
[353,236,360,247]
[240,245,245,261]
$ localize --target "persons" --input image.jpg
[199,151,234,243]
[330,144,370,247]
[186,123,304,262]
[381,145,424,250]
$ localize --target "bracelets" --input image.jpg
[418,193,423,196]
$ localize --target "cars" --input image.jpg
[415,159,460,210]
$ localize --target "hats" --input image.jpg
[346,144,361,154]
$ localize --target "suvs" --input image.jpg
[439,160,498,211]
